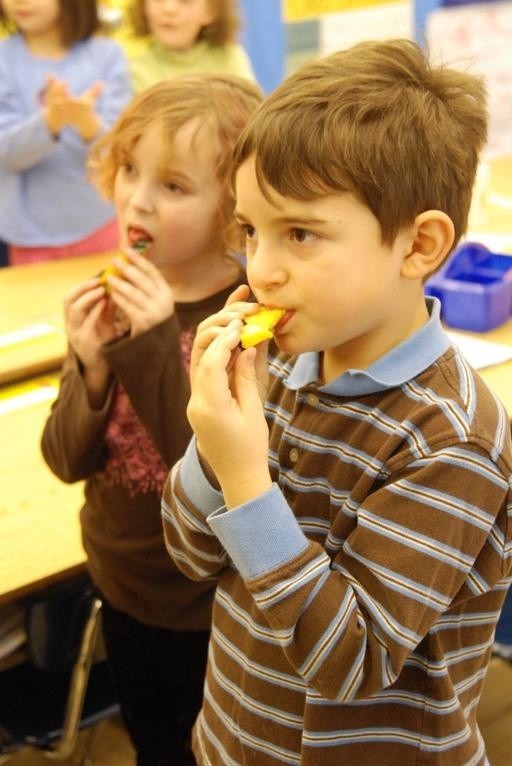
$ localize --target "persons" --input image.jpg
[117,0,261,95]
[164,31,512,764]
[2,1,132,259]
[38,72,267,764]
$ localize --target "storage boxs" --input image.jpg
[423,244,512,331]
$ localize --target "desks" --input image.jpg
[1,251,125,387]
[1,327,512,762]
